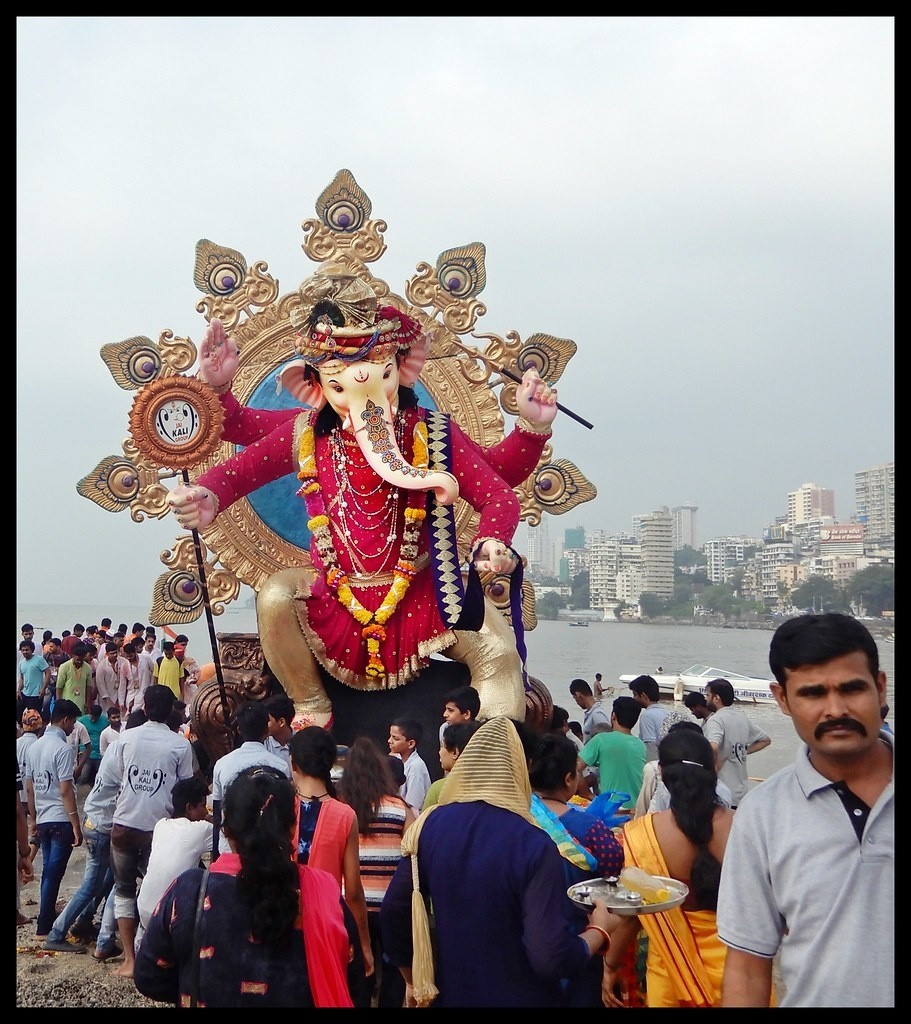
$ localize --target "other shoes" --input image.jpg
[37,914,59,924]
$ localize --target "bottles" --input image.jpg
[620,866,671,903]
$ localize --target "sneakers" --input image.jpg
[68,923,101,939]
[42,938,85,954]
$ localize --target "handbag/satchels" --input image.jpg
[586,791,630,828]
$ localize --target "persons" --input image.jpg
[529,675,772,877]
[287,727,375,977]
[602,729,776,1007]
[337,685,483,906]
[133,765,356,1007]
[165,318,558,721]
[16,618,201,721]
[593,673,609,694]
[16,684,193,978]
[378,716,629,1008]
[137,778,232,931]
[716,613,895,1007]
[211,694,296,862]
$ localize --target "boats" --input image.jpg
[619,663,780,705]
[569,620,589,627]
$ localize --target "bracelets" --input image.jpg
[585,925,611,955]
[603,956,620,968]
[19,847,31,857]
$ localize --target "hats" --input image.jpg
[174,644,184,656]
[22,710,43,731]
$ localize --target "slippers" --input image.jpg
[91,944,122,961]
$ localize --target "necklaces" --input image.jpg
[539,797,567,805]
